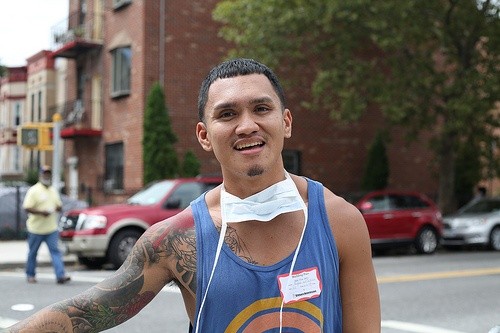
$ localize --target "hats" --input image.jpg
[40,165,51,173]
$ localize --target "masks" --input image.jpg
[220,177,308,225]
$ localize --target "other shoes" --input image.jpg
[27,278,36,283]
[57,277,71,283]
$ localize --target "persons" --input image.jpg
[0,58,381,333]
[22,165,72,283]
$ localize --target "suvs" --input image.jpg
[61,178,224,268]
[355,191,443,255]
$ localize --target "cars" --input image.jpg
[444,196,500,251]
